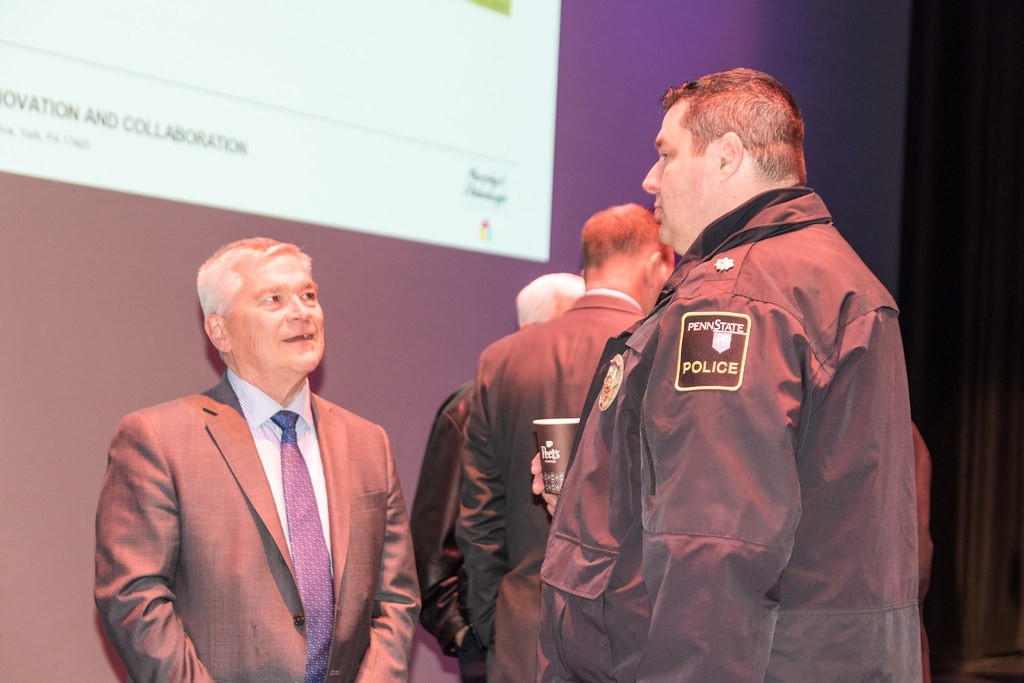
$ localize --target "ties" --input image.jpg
[269,409,335,683]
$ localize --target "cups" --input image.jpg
[533,418,580,495]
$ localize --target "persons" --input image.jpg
[532,67,925,683]
[411,203,935,683]
[93,238,422,683]
